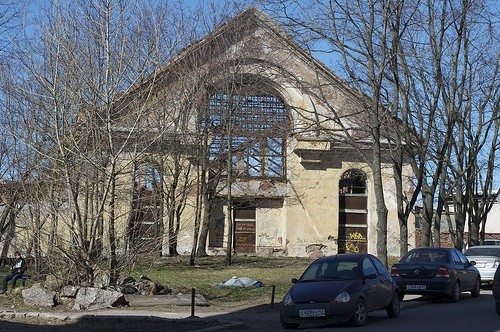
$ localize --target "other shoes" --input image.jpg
[0,287,6,294]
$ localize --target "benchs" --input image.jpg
[0,274,31,288]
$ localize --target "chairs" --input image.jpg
[421,256,431,261]
[324,267,337,277]
[351,266,366,276]
[435,256,446,262]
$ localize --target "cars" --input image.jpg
[391,247,482,302]
[463,246,500,291]
[279,254,401,329]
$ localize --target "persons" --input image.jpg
[0,251,27,294]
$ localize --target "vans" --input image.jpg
[463,239,500,246]
